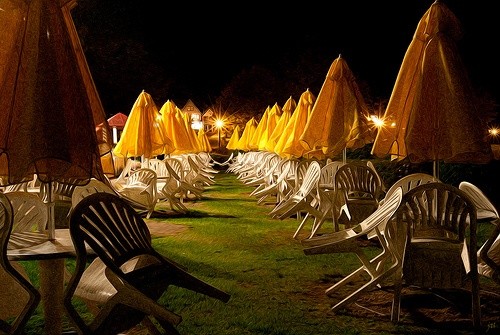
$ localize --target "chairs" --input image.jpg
[0,152,500,335]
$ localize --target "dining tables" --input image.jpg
[392,209,496,290]
[318,184,370,232]
[6,232,135,335]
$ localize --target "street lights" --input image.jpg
[214,120,223,149]
